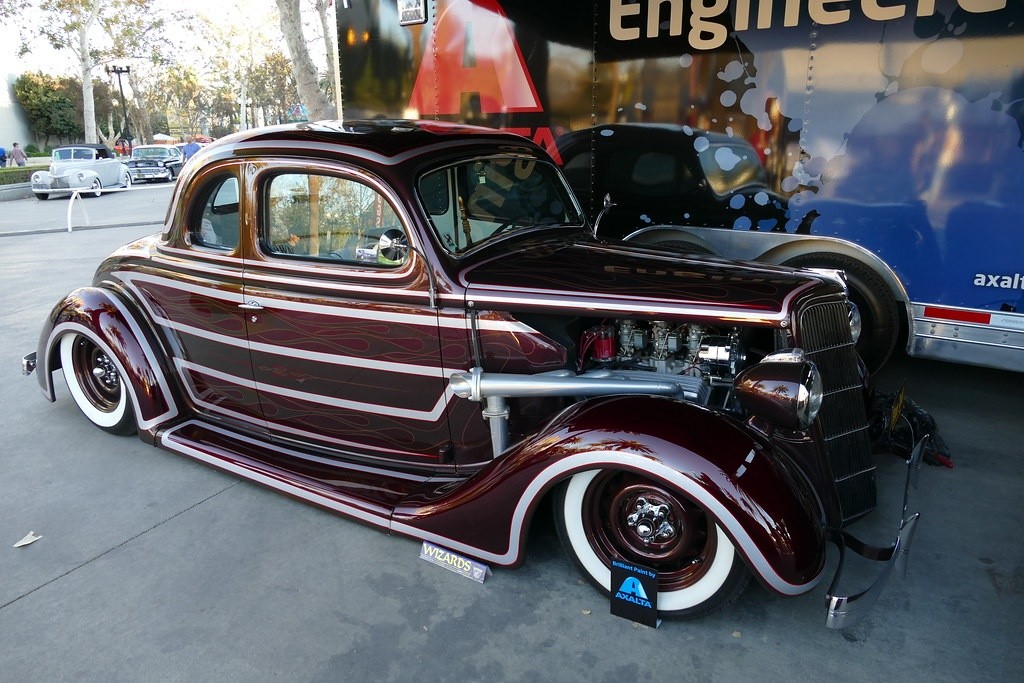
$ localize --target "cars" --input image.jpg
[20,115,932,648]
[496,123,814,240]
[113,139,138,156]
[124,143,186,185]
[28,143,134,201]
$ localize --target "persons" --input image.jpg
[182,135,201,166]
[0,142,29,167]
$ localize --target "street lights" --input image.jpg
[105,64,133,157]
